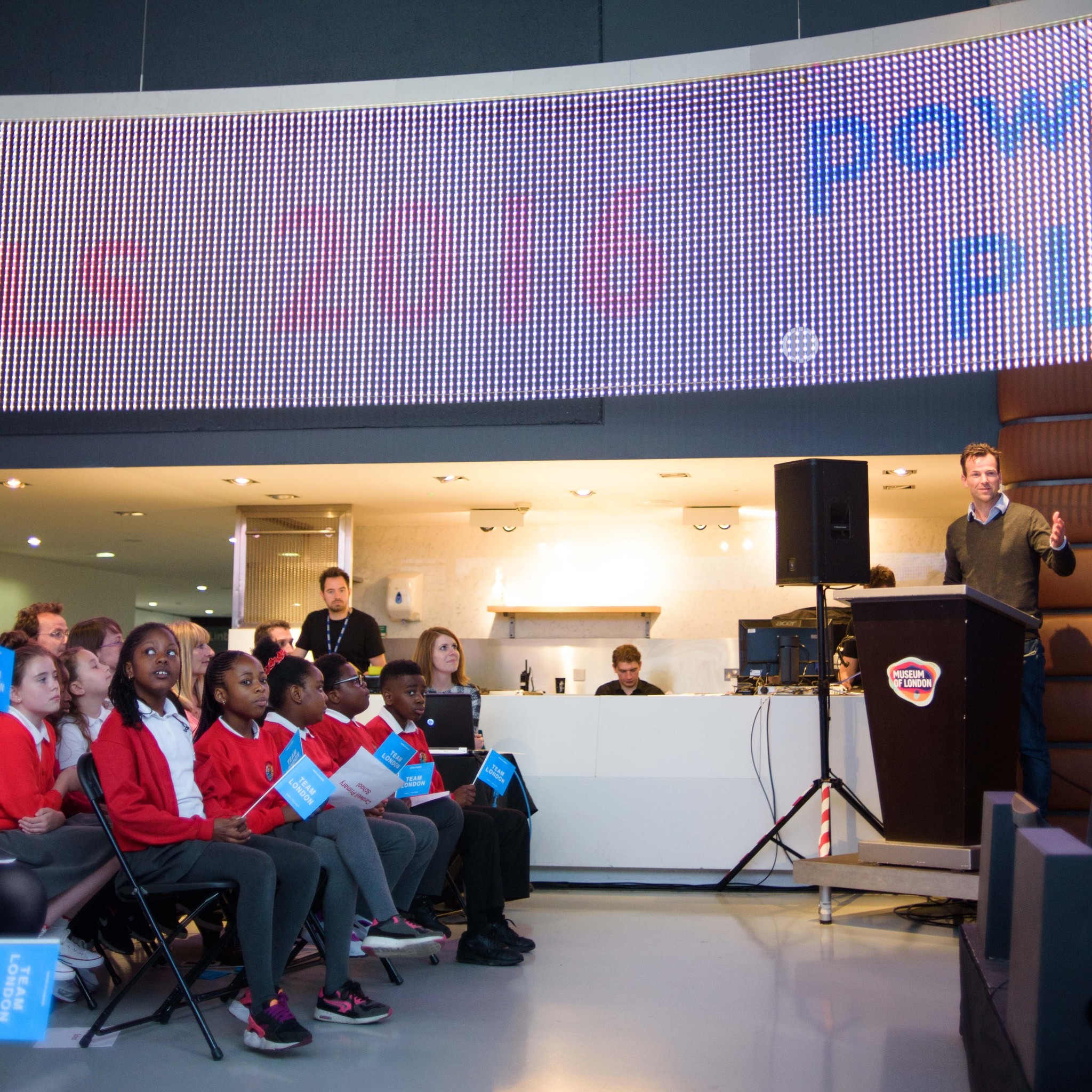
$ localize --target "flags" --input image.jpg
[279,728,303,776]
[274,755,335,820]
[0,943,60,1041]
[395,762,434,799]
[0,646,15,713]
[477,748,516,796]
[372,731,418,775]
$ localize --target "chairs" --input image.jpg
[75,753,441,1061]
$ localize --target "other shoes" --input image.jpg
[202,942,241,963]
[176,903,224,931]
[155,913,188,939]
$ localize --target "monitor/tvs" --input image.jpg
[738,619,834,687]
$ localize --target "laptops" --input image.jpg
[417,693,475,751]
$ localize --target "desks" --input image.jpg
[436,753,535,904]
[479,694,878,898]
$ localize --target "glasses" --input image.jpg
[35,630,70,640]
[99,639,125,650]
[332,674,364,686]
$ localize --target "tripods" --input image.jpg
[717,585,887,924]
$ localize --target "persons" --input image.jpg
[292,566,386,674]
[0,602,124,1002]
[415,627,484,750]
[269,803,274,809]
[838,566,895,691]
[306,654,451,940]
[366,660,536,966]
[170,619,445,1025]
[91,622,322,1048]
[595,644,665,695]
[943,442,1076,828]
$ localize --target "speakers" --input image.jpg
[776,457,870,586]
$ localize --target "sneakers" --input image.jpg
[75,968,100,993]
[489,919,536,952]
[361,914,447,958]
[352,914,373,941]
[428,893,462,914]
[301,911,368,958]
[54,960,76,981]
[97,902,135,955]
[228,985,289,1024]
[456,931,524,966]
[52,980,81,1003]
[397,894,451,938]
[57,929,104,969]
[244,1002,312,1051]
[119,909,156,942]
[314,980,392,1024]
